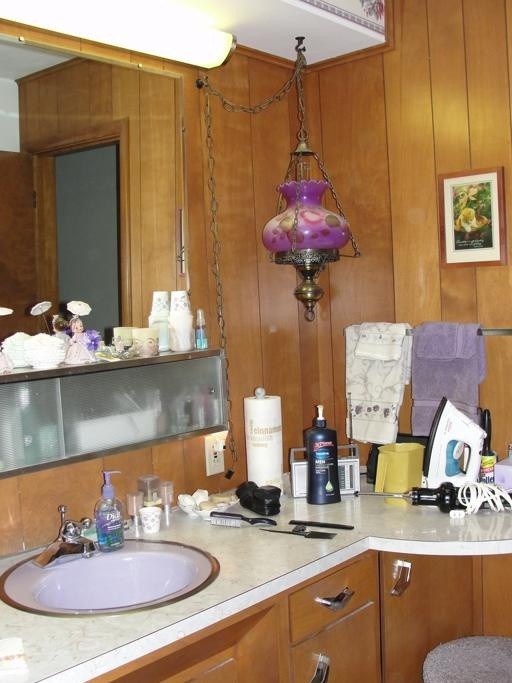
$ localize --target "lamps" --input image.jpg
[195,36,361,478]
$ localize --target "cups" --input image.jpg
[112,327,132,351]
[146,289,195,353]
[140,508,162,533]
[132,327,160,355]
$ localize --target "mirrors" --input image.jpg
[0,34,190,377]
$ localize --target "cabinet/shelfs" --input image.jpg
[287,555,380,682]
[113,607,280,682]
[0,347,226,479]
[380,550,512,683]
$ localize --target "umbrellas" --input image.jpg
[67,300,92,318]
[30,300,53,336]
[0,306,14,316]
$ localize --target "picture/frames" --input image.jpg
[438,166,508,266]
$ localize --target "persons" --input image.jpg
[63,316,95,364]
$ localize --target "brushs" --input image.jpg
[211,512,276,528]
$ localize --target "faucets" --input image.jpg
[30,504,97,567]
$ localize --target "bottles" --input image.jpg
[18,384,43,465]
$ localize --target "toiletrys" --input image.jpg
[195,308,207,349]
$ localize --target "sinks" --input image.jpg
[1,538,220,616]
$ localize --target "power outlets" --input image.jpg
[205,430,225,477]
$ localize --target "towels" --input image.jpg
[345,320,486,445]
